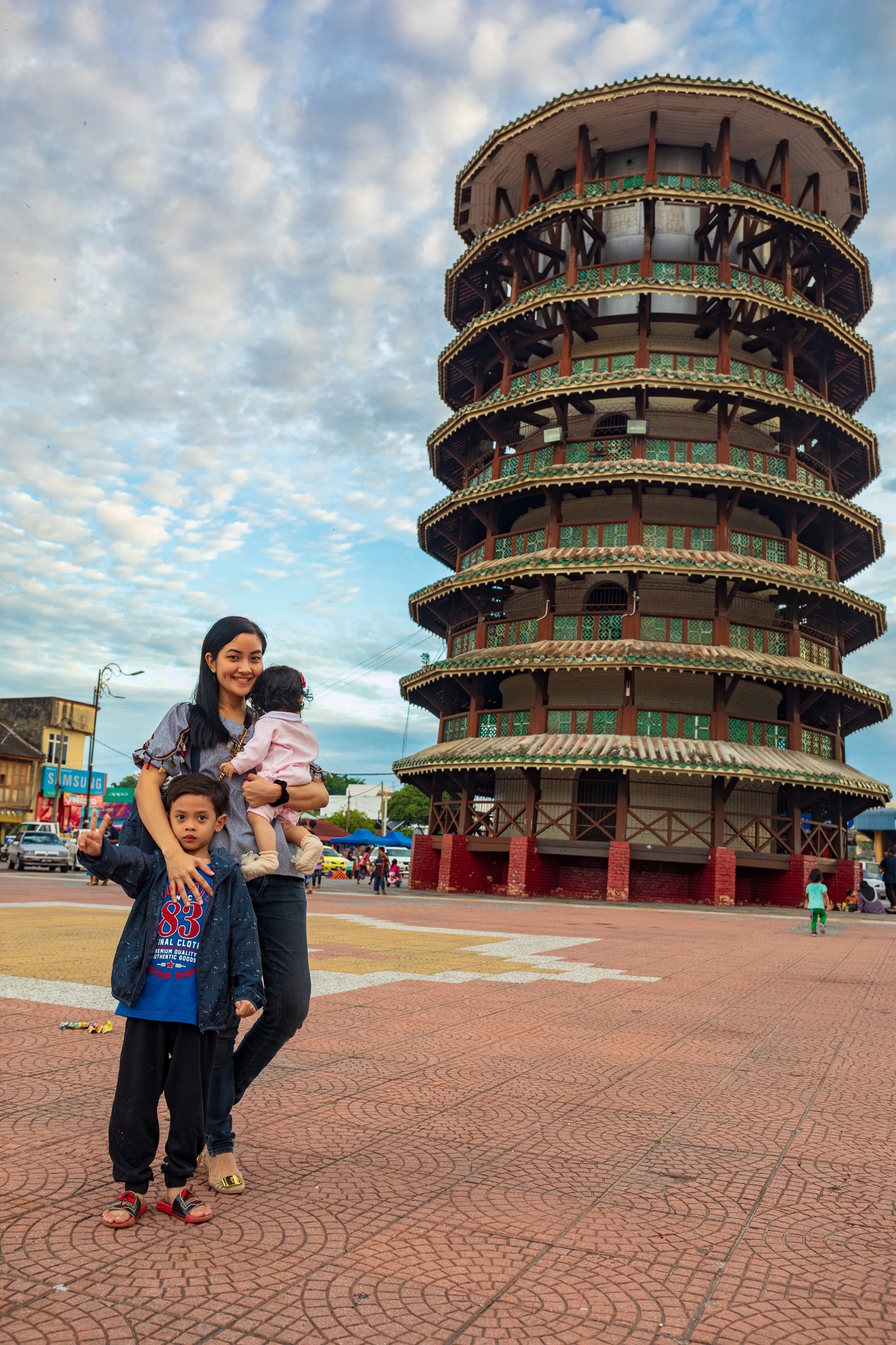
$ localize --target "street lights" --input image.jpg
[82,663,146,829]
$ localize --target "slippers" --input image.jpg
[156,1188,214,1223]
[101,1190,148,1228]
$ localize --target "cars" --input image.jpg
[6,830,71,872]
[368,846,411,877]
[65,829,119,871]
[862,869,888,899]
[321,845,351,876]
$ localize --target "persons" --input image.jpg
[132,615,329,1195]
[296,819,325,895]
[389,859,403,884]
[878,846,896,909]
[357,847,373,884]
[77,775,266,1230]
[805,868,832,937]
[86,815,120,887]
[366,853,392,884]
[845,881,887,914]
[220,666,324,880]
[368,847,389,895]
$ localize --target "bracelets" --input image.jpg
[269,779,290,808]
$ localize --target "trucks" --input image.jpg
[4,822,74,849]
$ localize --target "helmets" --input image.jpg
[350,845,355,850]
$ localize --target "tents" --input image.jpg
[331,829,412,847]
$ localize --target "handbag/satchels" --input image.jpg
[847,903,858,912]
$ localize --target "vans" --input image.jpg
[859,861,883,877]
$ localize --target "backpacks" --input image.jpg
[118,703,201,899]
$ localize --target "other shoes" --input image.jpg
[382,890,387,895]
[304,884,308,888]
[368,881,371,884]
[357,881,360,884]
[359,873,364,880]
[819,922,826,934]
[375,892,380,895]
[811,931,818,937]
[208,1153,245,1193]
[306,891,312,894]
